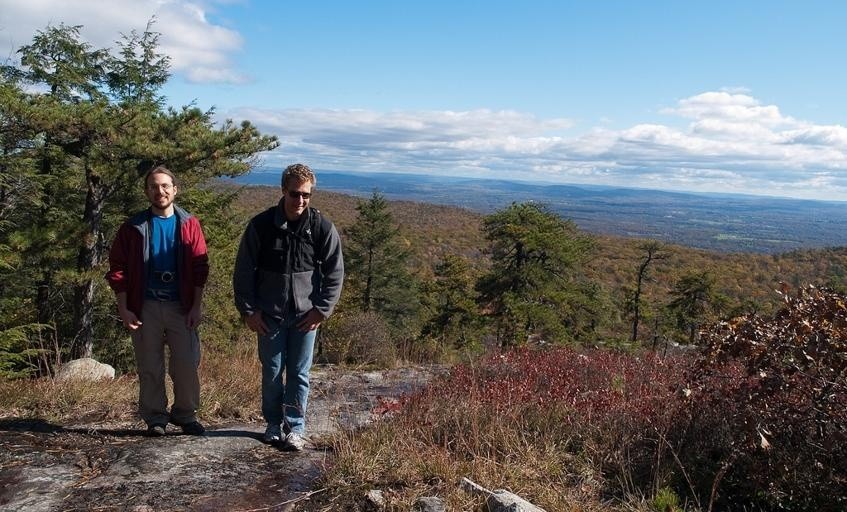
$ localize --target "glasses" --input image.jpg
[285,187,313,199]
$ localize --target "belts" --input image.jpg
[144,288,179,302]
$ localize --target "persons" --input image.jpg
[105,167,211,440]
[232,163,344,455]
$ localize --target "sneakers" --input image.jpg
[262,421,281,443]
[166,414,204,434]
[146,424,166,436]
[283,432,306,452]
